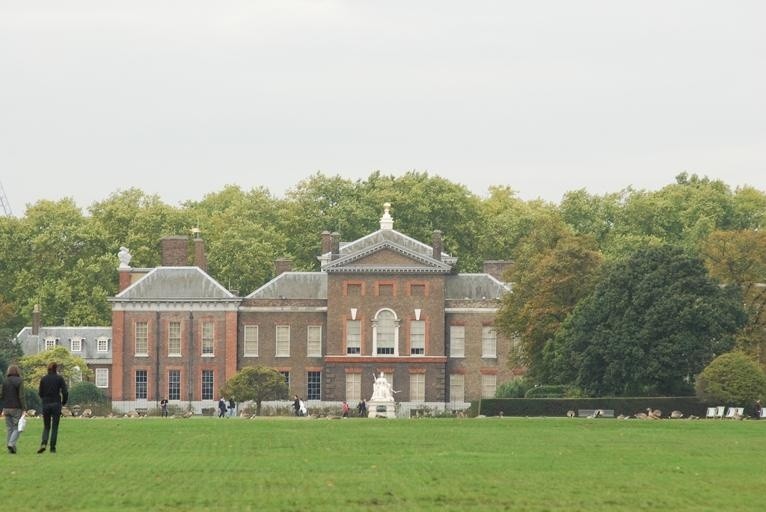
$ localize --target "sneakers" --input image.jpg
[37,447,45,453]
[8,444,16,453]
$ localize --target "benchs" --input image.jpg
[578,409,615,418]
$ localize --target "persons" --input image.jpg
[217,396,227,419]
[226,396,236,416]
[746,400,763,420]
[0,365,28,454]
[341,401,349,417]
[356,401,362,413]
[298,396,307,417]
[357,398,368,418]
[290,395,301,416]
[374,371,391,400]
[160,399,169,418]
[36,361,69,455]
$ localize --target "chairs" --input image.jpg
[705,405,766,420]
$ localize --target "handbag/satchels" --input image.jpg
[18,416,26,431]
